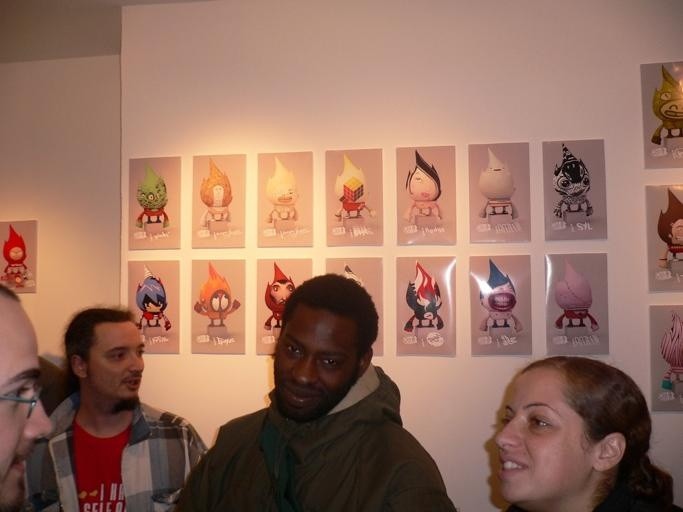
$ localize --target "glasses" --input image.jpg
[0,383,43,418]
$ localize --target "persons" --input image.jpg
[174,274,458,512]
[494,356,683,512]
[23,306,209,512]
[0,285,53,512]
